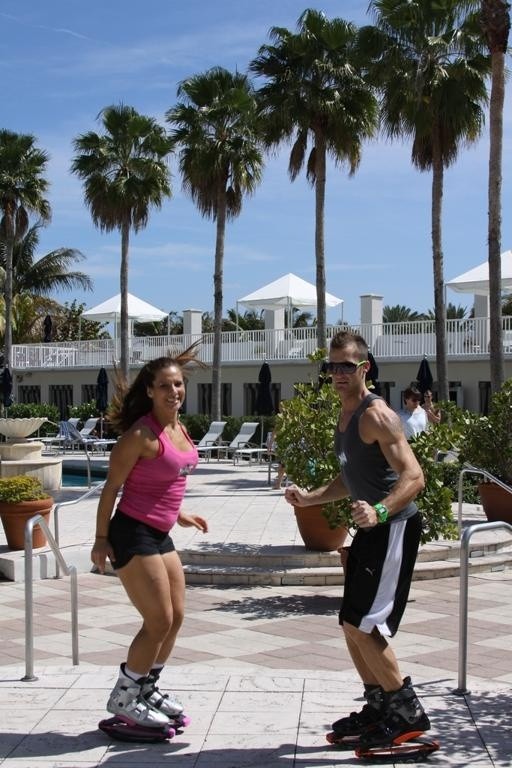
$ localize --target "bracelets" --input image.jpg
[96,535,107,539]
[374,502,388,523]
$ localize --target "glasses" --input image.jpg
[326,361,367,375]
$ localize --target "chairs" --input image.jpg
[192,422,277,466]
[42,418,118,457]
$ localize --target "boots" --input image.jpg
[106,663,170,729]
[362,676,431,745]
[143,667,185,717]
[332,682,386,734]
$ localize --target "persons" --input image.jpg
[395,385,427,441]
[420,389,441,425]
[267,398,304,490]
[285,329,431,748]
[59,400,120,451]
[91,334,209,731]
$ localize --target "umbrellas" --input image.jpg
[257,354,274,448]
[415,353,433,406]
[2,363,12,443]
[95,364,109,452]
[366,344,380,395]
[43,312,53,342]
[318,359,333,391]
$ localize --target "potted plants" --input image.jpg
[453,380,512,524]
[274,347,375,550]
[0,402,48,442]
[0,474,54,549]
